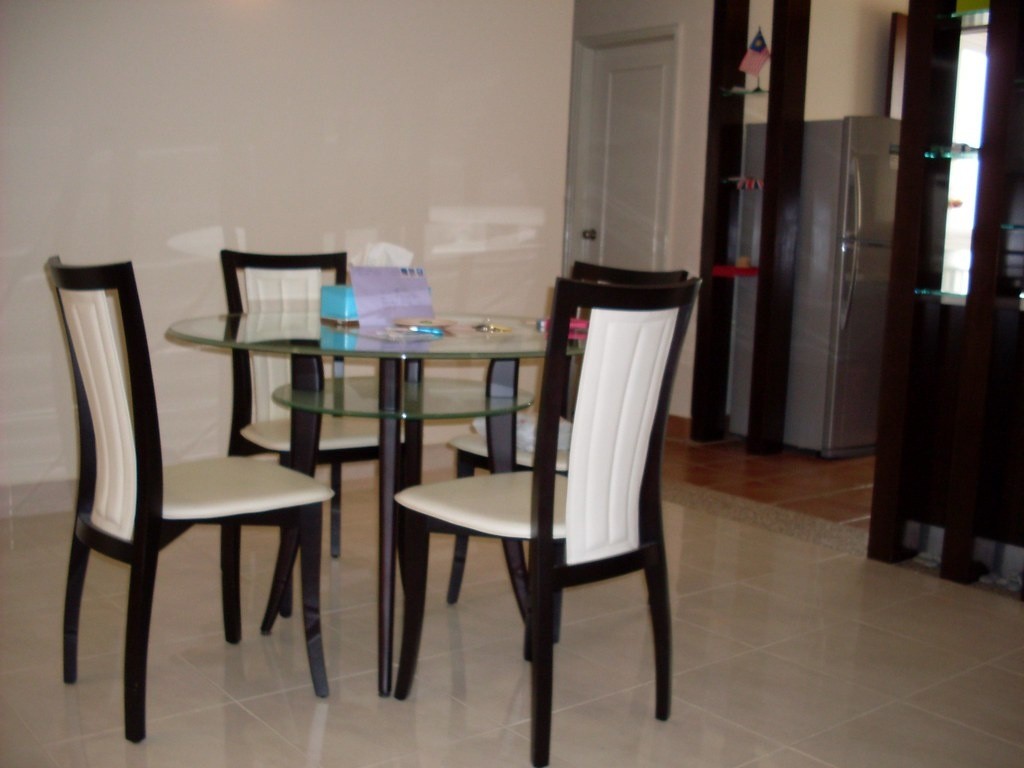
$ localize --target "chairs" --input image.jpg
[220,250,405,615]
[393,276,703,768]
[43,256,336,741]
[447,260,690,604]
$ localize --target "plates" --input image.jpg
[394,319,458,331]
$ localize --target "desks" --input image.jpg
[165,303,583,695]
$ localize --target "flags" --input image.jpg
[739,29,770,78]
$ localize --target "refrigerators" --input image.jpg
[727,114,905,462]
